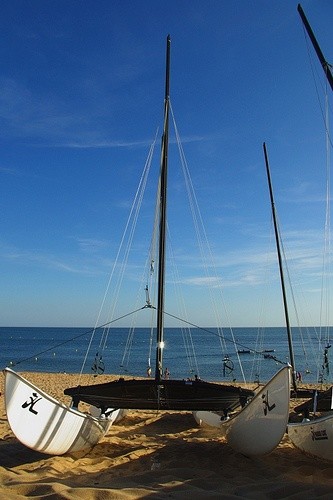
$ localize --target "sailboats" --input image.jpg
[64,26,254,410]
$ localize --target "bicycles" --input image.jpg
[224,353,235,375]
[91,352,107,375]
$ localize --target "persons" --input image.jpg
[146,367,170,380]
[58,370,67,375]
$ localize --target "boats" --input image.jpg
[287,415,333,460]
[89,405,120,424]
[219,364,292,458]
[1,367,122,459]
[192,411,230,429]
